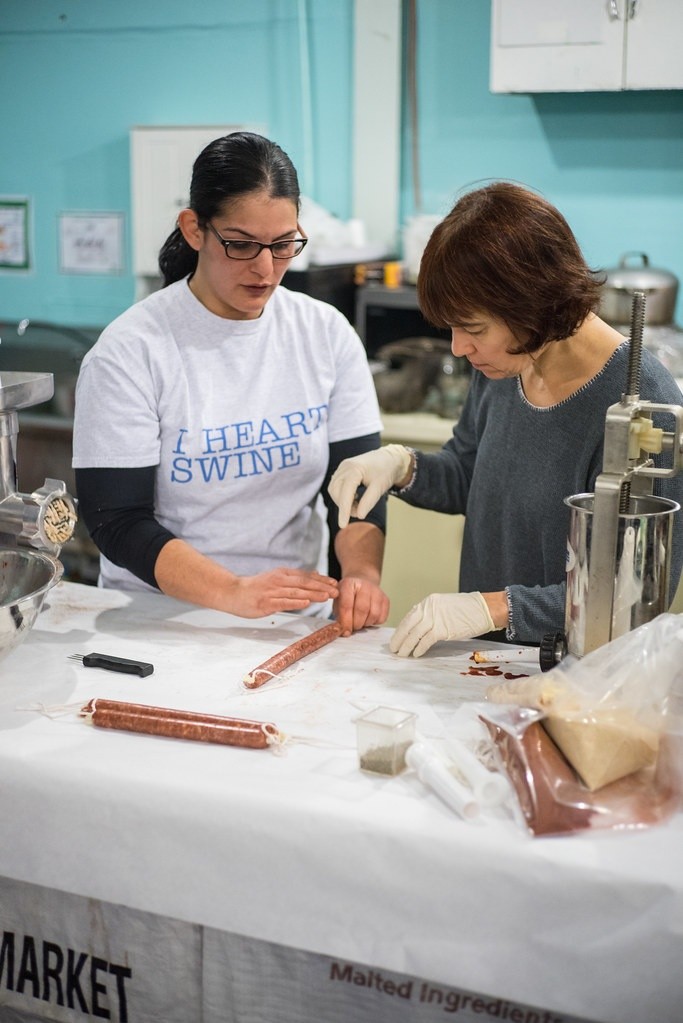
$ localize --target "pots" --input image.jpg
[586,251,678,327]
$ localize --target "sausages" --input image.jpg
[83,617,345,749]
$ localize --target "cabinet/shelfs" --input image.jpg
[131,124,268,278]
[487,0,683,96]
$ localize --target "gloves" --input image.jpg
[383,591,495,656]
[327,444,412,529]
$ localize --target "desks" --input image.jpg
[1,580,683,1023]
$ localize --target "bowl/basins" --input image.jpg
[0,547,66,660]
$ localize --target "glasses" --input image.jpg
[206,219,309,260]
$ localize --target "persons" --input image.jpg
[327,180,683,659]
[70,133,392,636]
